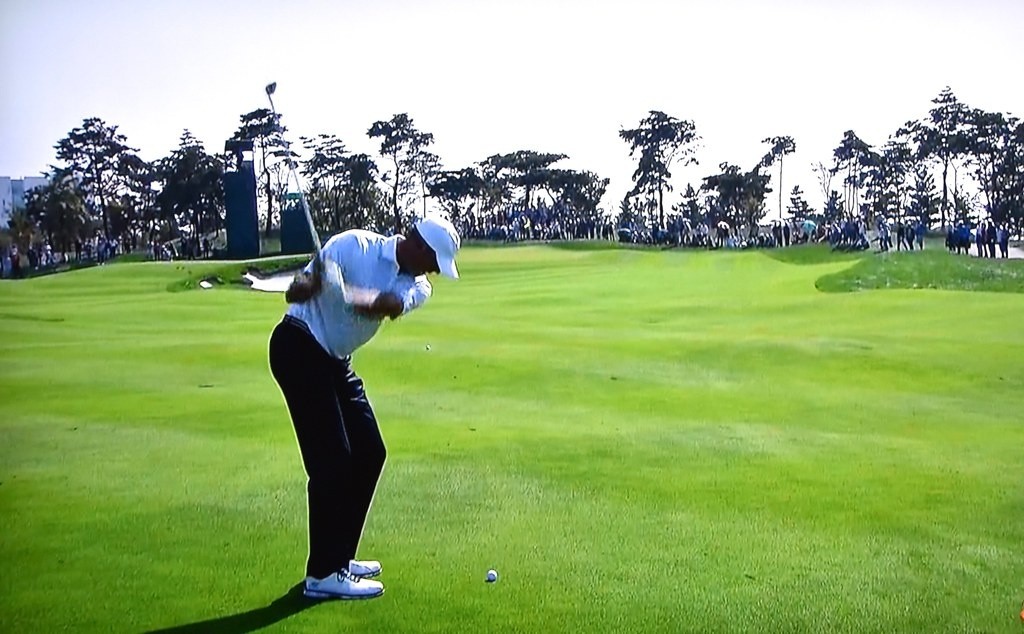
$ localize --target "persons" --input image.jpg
[0,206,1010,280]
[267,216,460,599]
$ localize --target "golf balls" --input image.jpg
[486,570,498,582]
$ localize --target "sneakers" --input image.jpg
[349,560,382,578]
[303,567,385,599]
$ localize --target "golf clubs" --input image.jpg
[265,81,324,275]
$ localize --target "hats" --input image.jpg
[416,216,461,280]
